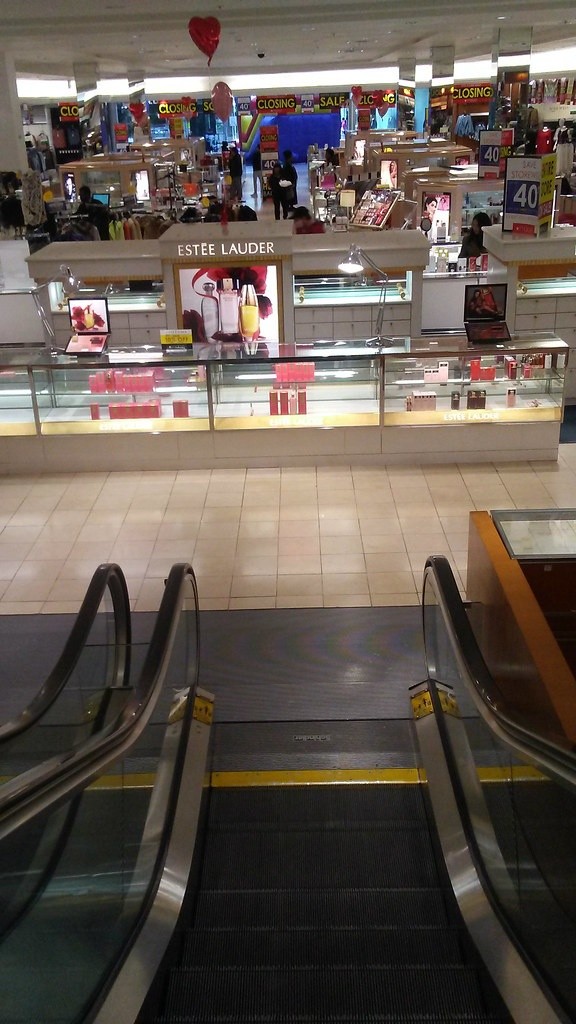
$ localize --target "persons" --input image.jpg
[182,149,189,161]
[422,197,449,237]
[228,147,243,201]
[268,150,298,220]
[250,146,264,196]
[469,290,498,314]
[535,118,574,178]
[64,175,76,202]
[458,212,492,257]
[291,206,324,234]
[389,161,397,189]
[69,186,111,220]
[318,149,337,173]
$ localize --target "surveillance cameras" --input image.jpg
[257,52,265,59]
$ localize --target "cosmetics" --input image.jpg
[407,354,563,410]
[320,189,398,231]
[268,363,314,414]
[429,248,489,275]
[89,368,189,418]
[199,275,263,357]
[83,306,100,335]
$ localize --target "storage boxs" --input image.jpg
[270,362,315,416]
[413,354,530,410]
[85,371,189,420]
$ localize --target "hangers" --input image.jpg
[112,211,134,221]
[67,215,74,229]
[79,214,86,226]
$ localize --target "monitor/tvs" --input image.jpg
[92,193,110,207]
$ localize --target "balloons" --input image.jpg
[128,95,192,128]
[211,82,235,122]
[188,16,222,66]
[351,85,397,118]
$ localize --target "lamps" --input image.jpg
[340,189,356,219]
[29,265,85,356]
[337,243,395,348]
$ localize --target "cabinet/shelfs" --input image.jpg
[461,207,502,229]
[50,312,168,348]
[516,291,576,407]
[293,303,411,343]
[0,333,569,473]
